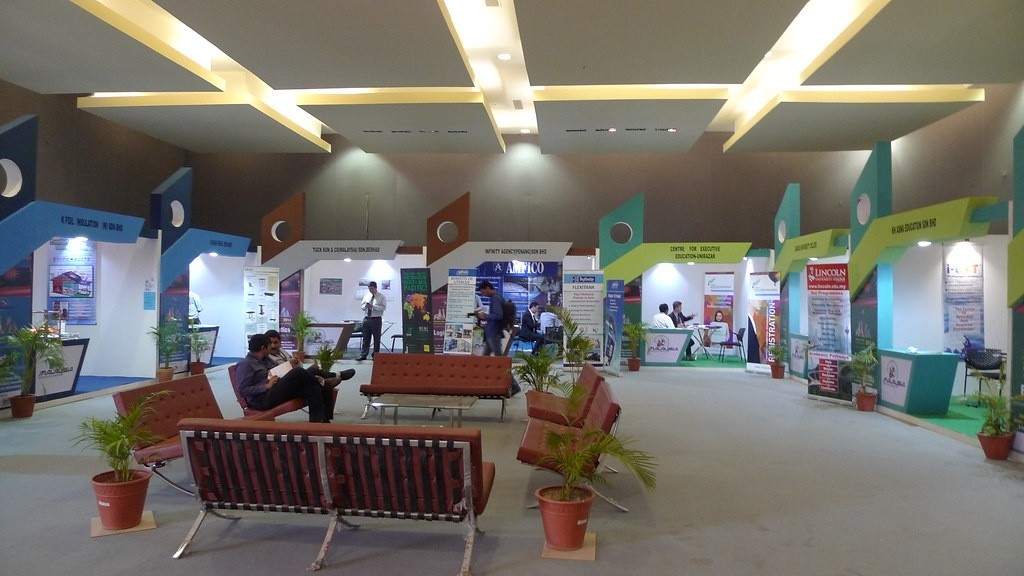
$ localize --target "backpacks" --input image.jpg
[496,294,517,330]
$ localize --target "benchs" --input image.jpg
[112,352,512,576]
[515,363,630,514]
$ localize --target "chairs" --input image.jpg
[342,319,363,351]
[719,328,747,363]
[964,347,1002,406]
[542,326,563,348]
[391,334,404,352]
[513,321,535,355]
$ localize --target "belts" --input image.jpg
[365,316,381,319]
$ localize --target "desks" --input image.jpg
[304,322,356,359]
[687,325,722,361]
[640,328,694,366]
[878,348,961,416]
[34,338,89,402]
[188,324,220,367]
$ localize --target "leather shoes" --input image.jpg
[355,356,366,361]
[372,357,373,361]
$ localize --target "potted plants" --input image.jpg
[512,341,566,395]
[963,359,1024,460]
[287,310,319,362]
[0,319,64,418]
[68,390,172,530]
[769,344,786,379]
[533,379,657,552]
[840,342,880,412]
[623,321,648,371]
[145,313,215,382]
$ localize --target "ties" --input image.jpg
[533,315,537,333]
[678,314,680,324]
[368,294,374,317]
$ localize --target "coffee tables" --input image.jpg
[368,392,479,427]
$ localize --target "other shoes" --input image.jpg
[510,386,521,397]
[531,351,538,358]
[686,357,695,361]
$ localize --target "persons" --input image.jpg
[714,311,726,322]
[474,294,483,328]
[748,300,762,363]
[474,281,522,397]
[234,330,356,424]
[652,303,675,328]
[355,281,387,362]
[519,302,543,356]
[668,300,697,361]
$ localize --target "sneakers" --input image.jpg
[323,377,341,391]
[309,419,330,423]
[340,369,355,381]
